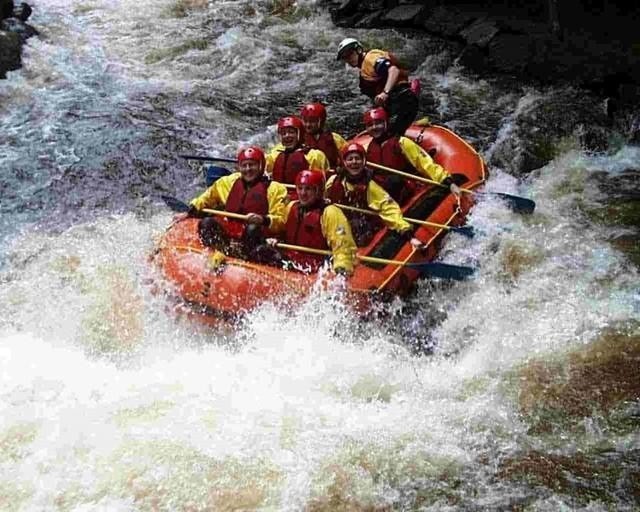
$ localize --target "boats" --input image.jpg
[152,118,490,330]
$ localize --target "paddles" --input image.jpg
[366,161,536,216]
[178,155,239,166]
[161,194,247,220]
[333,202,512,253]
[276,243,476,282]
[206,165,296,194]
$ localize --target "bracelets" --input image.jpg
[383,89,388,94]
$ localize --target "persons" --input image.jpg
[264,116,329,200]
[253,171,357,280]
[300,103,348,166]
[173,148,288,255]
[334,38,420,137]
[362,109,462,199]
[324,142,426,250]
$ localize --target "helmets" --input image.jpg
[342,143,366,167]
[363,108,388,132]
[301,103,326,128]
[330,38,363,60]
[295,169,325,199]
[278,116,304,142]
[238,146,266,173]
